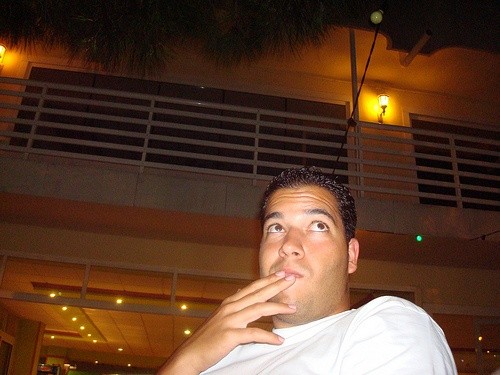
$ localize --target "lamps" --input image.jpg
[377,91,390,122]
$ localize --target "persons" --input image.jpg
[156,166,457,375]
